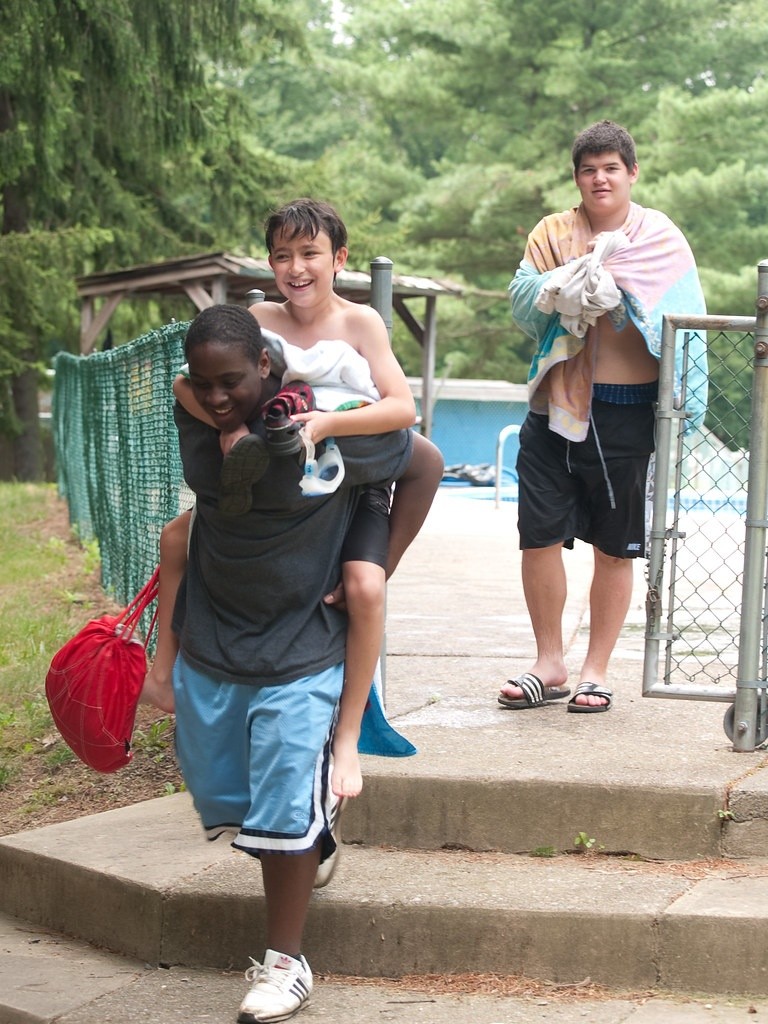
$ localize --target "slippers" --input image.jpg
[567,682,613,713]
[498,673,570,708]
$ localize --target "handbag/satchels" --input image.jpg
[45,569,165,775]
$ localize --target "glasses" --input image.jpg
[299,445,345,497]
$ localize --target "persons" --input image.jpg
[497,121,707,712]
[172,303,446,1024]
[138,198,416,798]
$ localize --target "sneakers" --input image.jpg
[313,754,348,888]
[238,949,313,1024]
[219,434,270,517]
[261,380,316,457]
